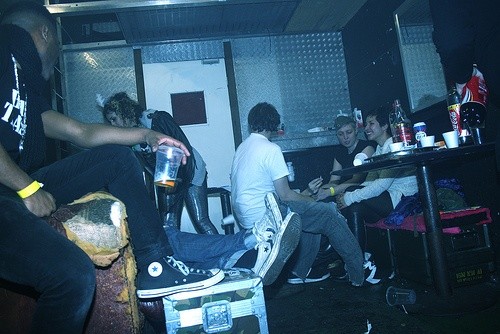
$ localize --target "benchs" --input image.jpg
[366,204,496,286]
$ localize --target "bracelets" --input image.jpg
[16,181,41,199]
[329,186,336,197]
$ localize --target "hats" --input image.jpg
[334,116,353,130]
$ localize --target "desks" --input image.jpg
[331,142,500,316]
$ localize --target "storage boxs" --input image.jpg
[161,267,269,334]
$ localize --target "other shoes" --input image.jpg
[351,260,394,287]
[287,268,330,284]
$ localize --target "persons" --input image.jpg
[162,193,302,288]
[299,177,374,279]
[102,92,219,236]
[315,115,375,229]
[341,107,420,240]
[0,1,224,334]
[232,102,397,286]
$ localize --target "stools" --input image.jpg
[207,186,236,236]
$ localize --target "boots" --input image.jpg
[315,211,368,282]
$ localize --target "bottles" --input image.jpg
[447,83,473,146]
[286,162,296,183]
[393,105,413,147]
[389,98,408,141]
[459,64,488,129]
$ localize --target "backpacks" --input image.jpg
[383,181,468,226]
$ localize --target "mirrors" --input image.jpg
[393,0,500,113]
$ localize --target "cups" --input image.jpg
[386,286,416,305]
[471,129,484,144]
[419,135,435,146]
[221,214,236,226]
[442,130,458,148]
[353,153,369,166]
[153,145,183,188]
[413,122,426,148]
[388,142,404,152]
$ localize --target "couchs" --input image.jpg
[0,189,163,334]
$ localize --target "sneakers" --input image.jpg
[135,254,225,298]
[250,211,302,286]
[251,191,284,240]
